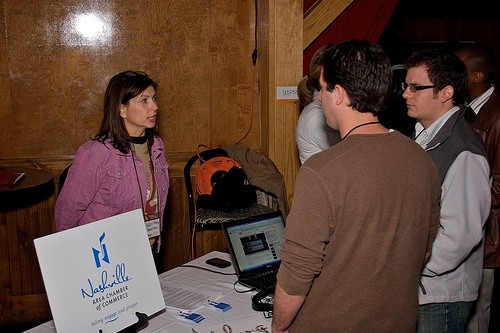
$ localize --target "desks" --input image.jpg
[0,166,55,211]
[22,251,273,333]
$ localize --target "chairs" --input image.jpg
[184,148,285,261]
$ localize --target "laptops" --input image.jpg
[221,210,286,297]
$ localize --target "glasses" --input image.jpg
[401,82,436,92]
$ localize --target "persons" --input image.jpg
[54,71,169,250]
[272,37,500,333]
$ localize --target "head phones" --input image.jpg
[252,285,276,311]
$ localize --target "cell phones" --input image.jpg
[206,258,231,268]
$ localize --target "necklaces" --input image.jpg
[129,136,148,144]
[340,122,380,141]
